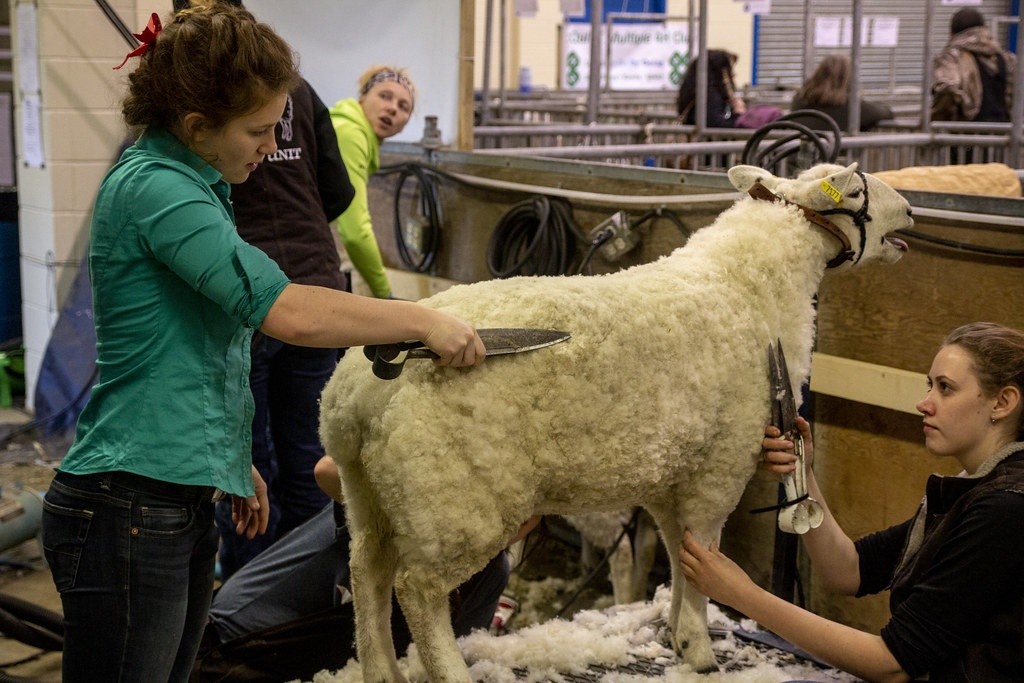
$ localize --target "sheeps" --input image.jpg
[316,161,915,683]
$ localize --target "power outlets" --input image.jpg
[588,209,640,262]
[404,214,431,255]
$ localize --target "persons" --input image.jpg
[678,321,1024,683]
[791,55,894,131]
[678,49,752,167]
[40,0,583,683]
[930,6,1019,165]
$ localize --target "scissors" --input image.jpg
[363,328,571,380]
[766,338,824,534]
